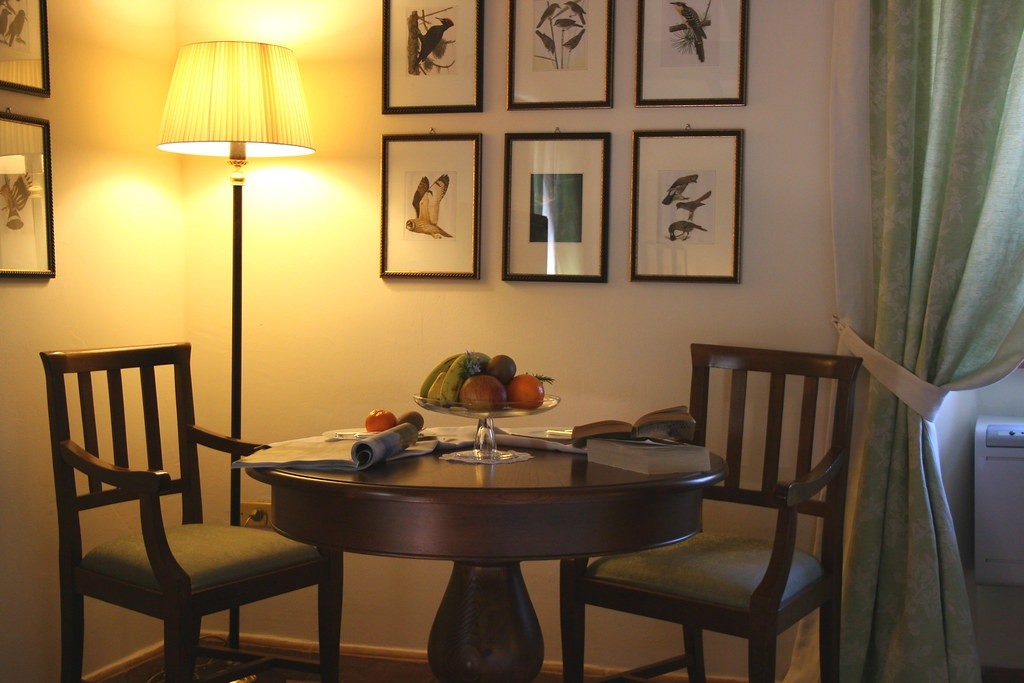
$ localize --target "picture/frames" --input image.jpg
[502,132,614,282]
[383,0,483,114]
[506,0,614,108]
[0,0,50,98]
[381,134,481,278]
[0,113,55,278]
[629,128,742,282]
[635,0,746,108]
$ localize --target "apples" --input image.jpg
[460,373,507,411]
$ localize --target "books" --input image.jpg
[571,405,696,445]
[231,422,419,472]
[586,437,711,476]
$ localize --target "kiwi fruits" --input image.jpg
[486,355,517,383]
[398,412,424,431]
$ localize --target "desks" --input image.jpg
[243,427,729,683]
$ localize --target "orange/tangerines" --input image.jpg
[506,374,544,410]
[365,409,398,433]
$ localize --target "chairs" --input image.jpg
[38,342,345,683]
[561,345,863,683]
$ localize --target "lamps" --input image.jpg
[155,39,317,662]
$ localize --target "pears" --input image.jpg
[427,372,446,404]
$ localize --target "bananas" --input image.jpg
[419,351,492,408]
